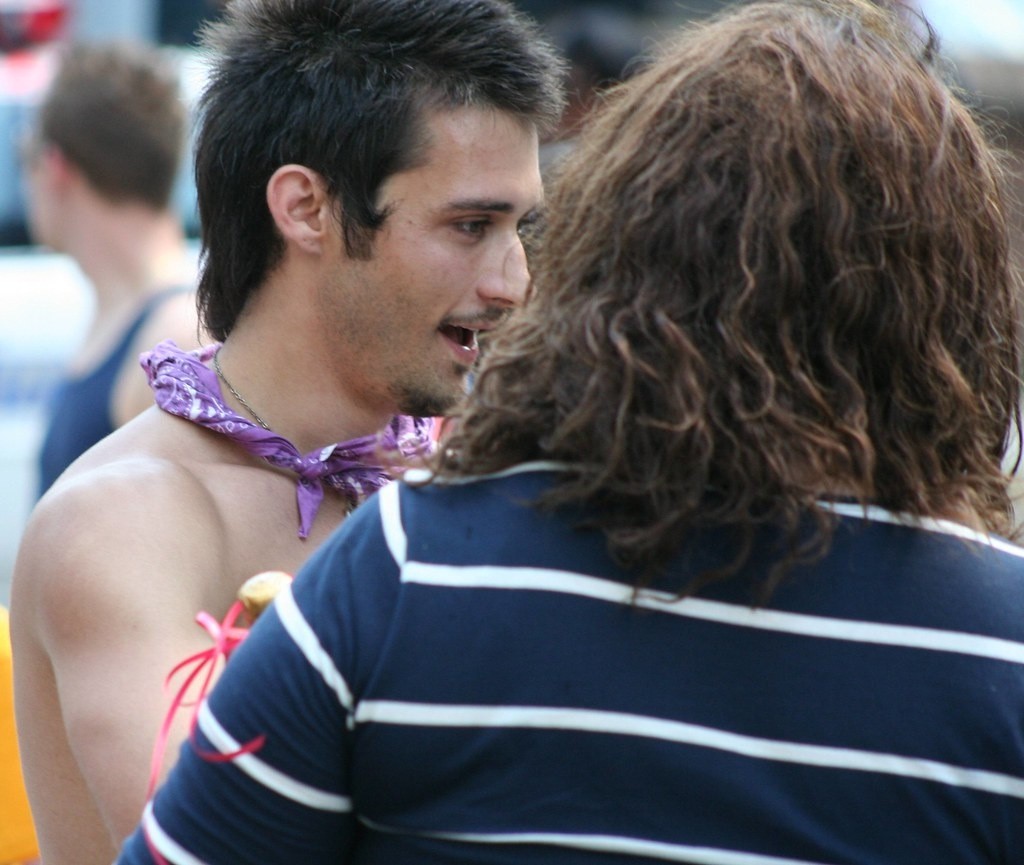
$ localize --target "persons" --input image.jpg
[9,2,1024,864]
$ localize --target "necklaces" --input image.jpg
[213,349,285,432]
